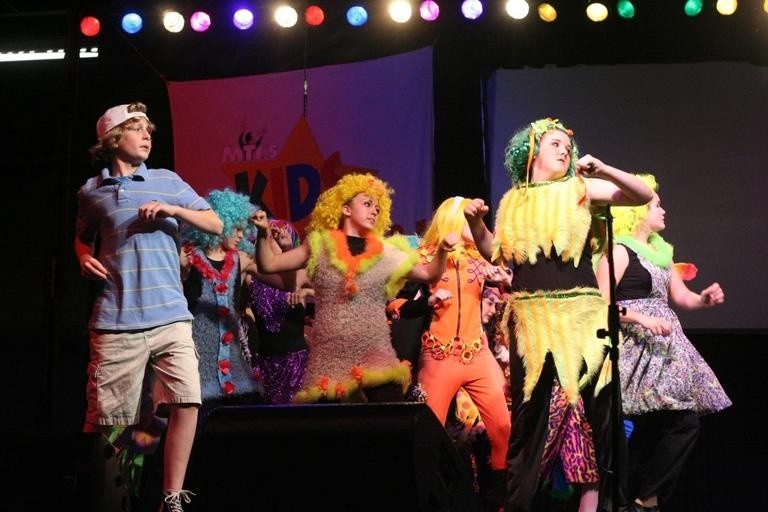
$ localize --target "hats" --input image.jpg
[96,104,149,137]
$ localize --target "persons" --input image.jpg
[462,119,731,510]
[75,104,224,512]
[176,173,513,511]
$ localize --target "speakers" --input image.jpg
[203,402,444,510]
